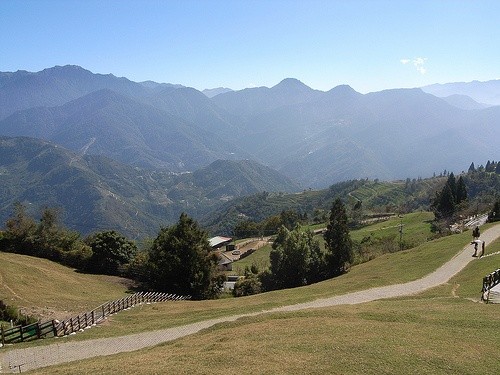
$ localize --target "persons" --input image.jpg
[474,242,478,256]
[482,241,485,256]
[471,225,480,241]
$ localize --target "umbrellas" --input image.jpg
[473,240,481,243]
